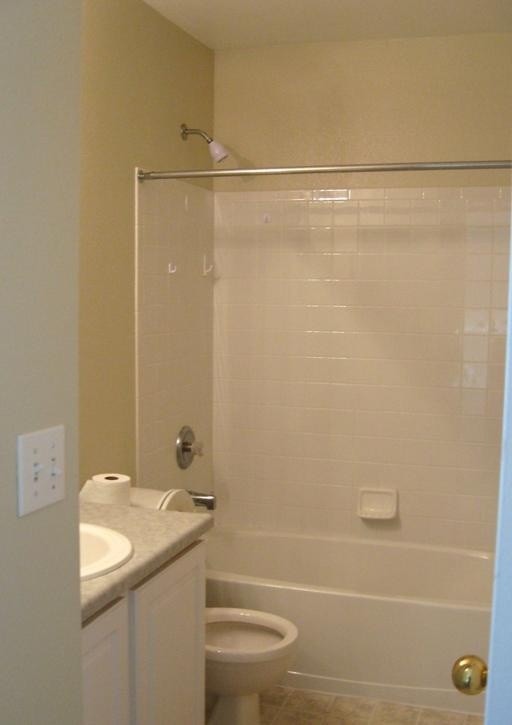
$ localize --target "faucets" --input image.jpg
[187,489,216,511]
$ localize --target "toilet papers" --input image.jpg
[80,472,131,504]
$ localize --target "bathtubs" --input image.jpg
[205,529,495,715]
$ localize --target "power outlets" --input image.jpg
[18,424,67,518]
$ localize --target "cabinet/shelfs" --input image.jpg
[76,541,206,725]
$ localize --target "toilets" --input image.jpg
[128,487,299,724]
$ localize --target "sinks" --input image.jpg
[80,522,134,581]
